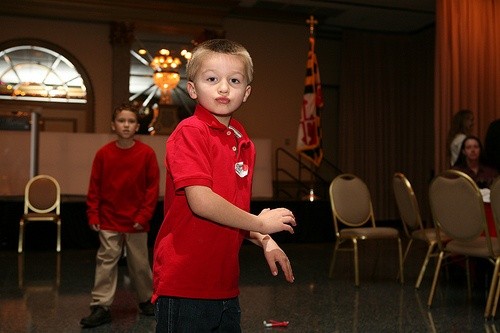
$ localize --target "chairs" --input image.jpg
[327,173,405,288]
[392,173,470,289]
[427,170,500,311]
[17,174,62,289]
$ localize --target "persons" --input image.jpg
[151,39,297,333]
[447,109,474,167]
[80,105,161,327]
[453,136,488,189]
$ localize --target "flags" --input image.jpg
[296,33,324,167]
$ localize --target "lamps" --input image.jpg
[127,37,191,115]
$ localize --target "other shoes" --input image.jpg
[139,302,157,316]
[79,306,112,327]
